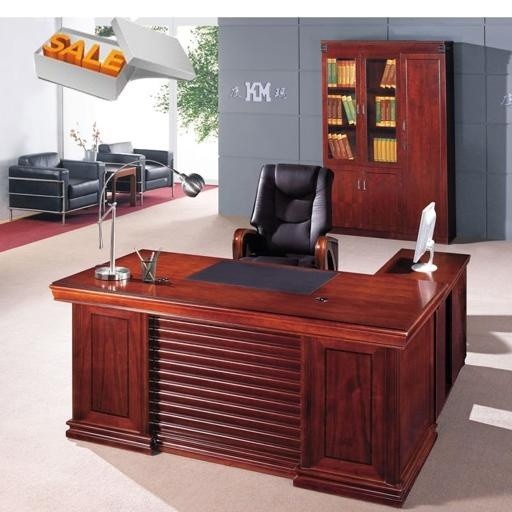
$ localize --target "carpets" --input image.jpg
[0,183,218,252]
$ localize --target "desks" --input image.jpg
[105,166,136,206]
[49,248,471,509]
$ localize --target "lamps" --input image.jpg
[94,159,206,282]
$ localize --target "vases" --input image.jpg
[85,152,96,163]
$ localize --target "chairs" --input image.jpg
[233,163,338,271]
[8,152,105,224]
[97,141,173,206]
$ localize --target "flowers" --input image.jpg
[69,120,104,152]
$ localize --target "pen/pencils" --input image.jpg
[133,246,163,280]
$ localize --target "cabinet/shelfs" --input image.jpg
[321,40,456,244]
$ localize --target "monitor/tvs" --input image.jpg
[418,272,438,309]
[411,202,437,272]
